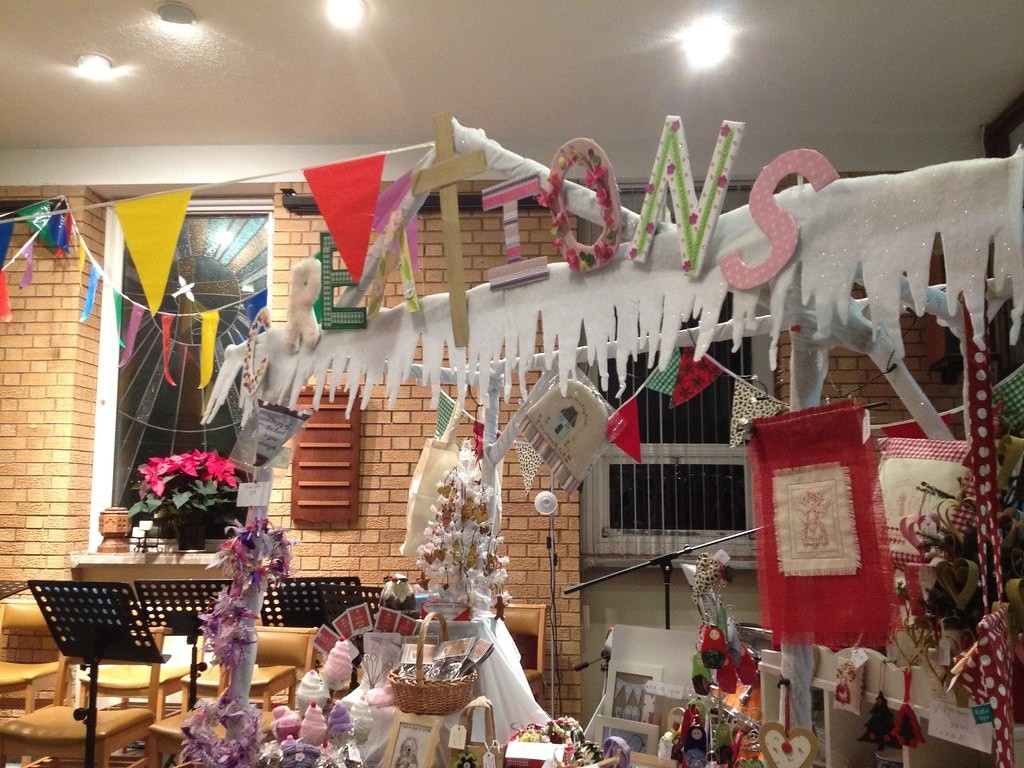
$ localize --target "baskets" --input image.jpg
[387,610,477,715]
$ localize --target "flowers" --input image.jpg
[127,448,245,520]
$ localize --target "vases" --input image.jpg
[174,513,210,553]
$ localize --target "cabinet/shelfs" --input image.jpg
[758,638,1023,768]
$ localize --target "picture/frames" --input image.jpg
[593,714,660,756]
[380,708,445,768]
[613,747,679,768]
[602,660,667,728]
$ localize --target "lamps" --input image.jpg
[155,2,198,33]
[76,52,116,81]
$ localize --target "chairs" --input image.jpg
[179,609,298,715]
[75,601,211,757]
[0,598,82,768]
[0,625,174,768]
[147,624,323,768]
[487,601,549,708]
